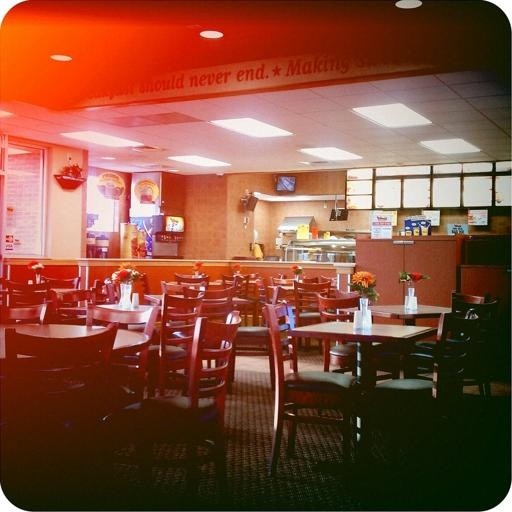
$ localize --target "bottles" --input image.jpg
[297,248,356,264]
[353,309,364,330]
[132,293,141,310]
[361,309,374,330]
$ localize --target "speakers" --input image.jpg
[247,196,258,212]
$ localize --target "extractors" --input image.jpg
[276,215,318,237]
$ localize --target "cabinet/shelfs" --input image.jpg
[351,235,462,340]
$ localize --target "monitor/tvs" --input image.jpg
[274,175,296,194]
[329,208,349,221]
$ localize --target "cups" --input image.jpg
[403,295,420,311]
